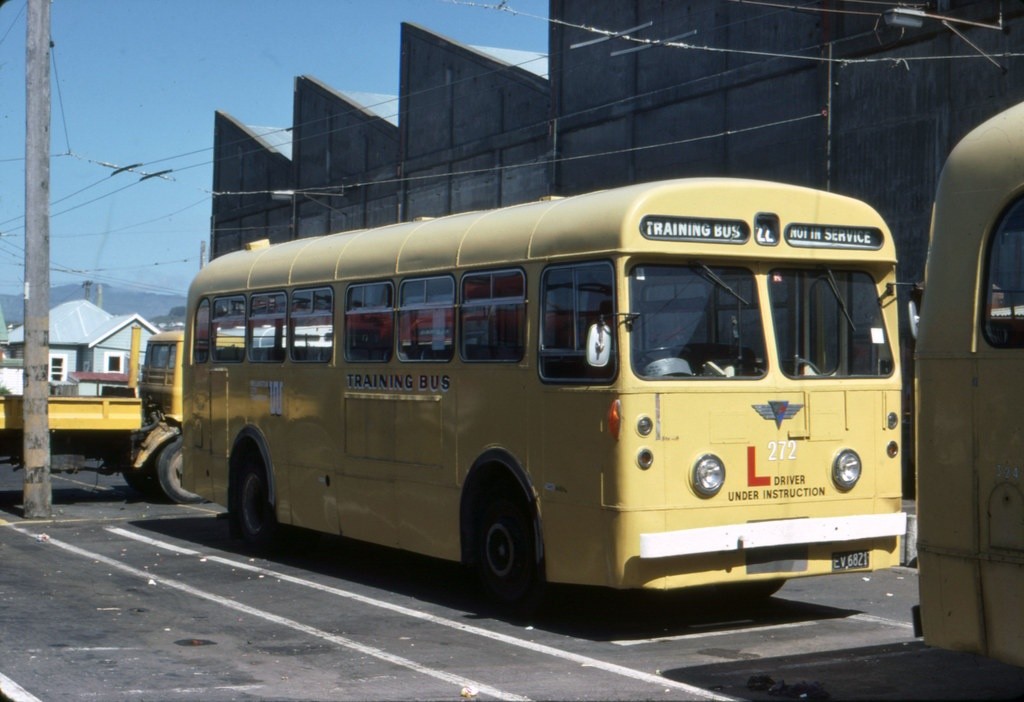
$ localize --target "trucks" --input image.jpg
[0,326,239,503]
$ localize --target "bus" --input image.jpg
[912,92,1023,702]
[180,178,910,624]
